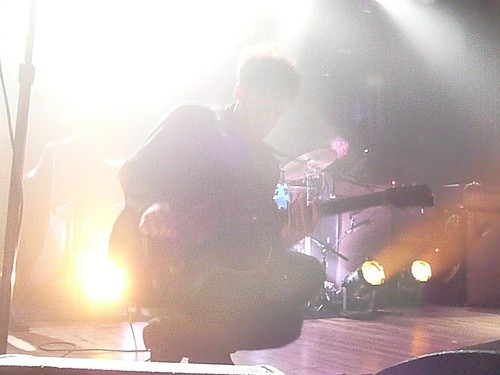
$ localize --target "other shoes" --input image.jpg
[142,314,186,362]
[187,336,236,365]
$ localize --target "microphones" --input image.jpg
[346,219,371,234]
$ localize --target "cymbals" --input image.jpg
[283,147,338,180]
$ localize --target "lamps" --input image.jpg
[336,260,385,316]
[388,259,434,305]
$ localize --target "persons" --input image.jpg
[106,55,336,364]
[429,183,493,309]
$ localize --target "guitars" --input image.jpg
[145,171,434,298]
